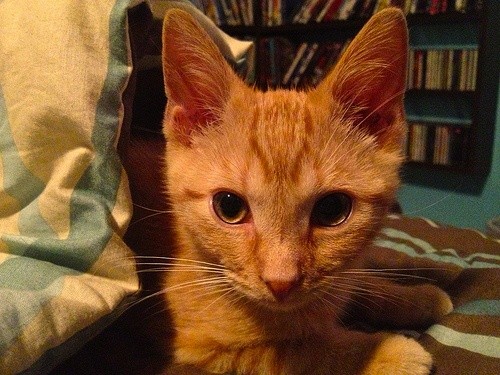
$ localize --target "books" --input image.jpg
[200,0,480,167]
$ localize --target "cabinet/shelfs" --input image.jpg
[191,0,499,234]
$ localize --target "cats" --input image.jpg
[118,7,452,375]
